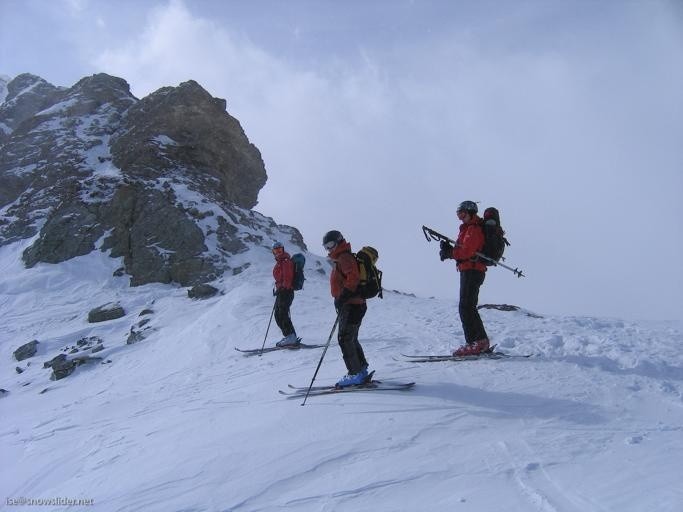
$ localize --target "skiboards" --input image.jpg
[234,344,327,357]
[279,382,416,395]
[392,353,534,362]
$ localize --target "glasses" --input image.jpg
[457,211,466,220]
[323,241,336,250]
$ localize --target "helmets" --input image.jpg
[456,201,478,213]
[272,240,284,248]
[322,230,343,246]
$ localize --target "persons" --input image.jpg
[272,241,299,346]
[439,200,490,356]
[322,230,370,388]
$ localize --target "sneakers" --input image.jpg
[335,368,369,386]
[276,332,299,346]
[453,339,490,356]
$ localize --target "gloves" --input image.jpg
[334,288,354,307]
[439,238,453,261]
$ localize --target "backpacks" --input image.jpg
[292,253,306,290]
[481,207,511,266]
[357,246,383,300]
